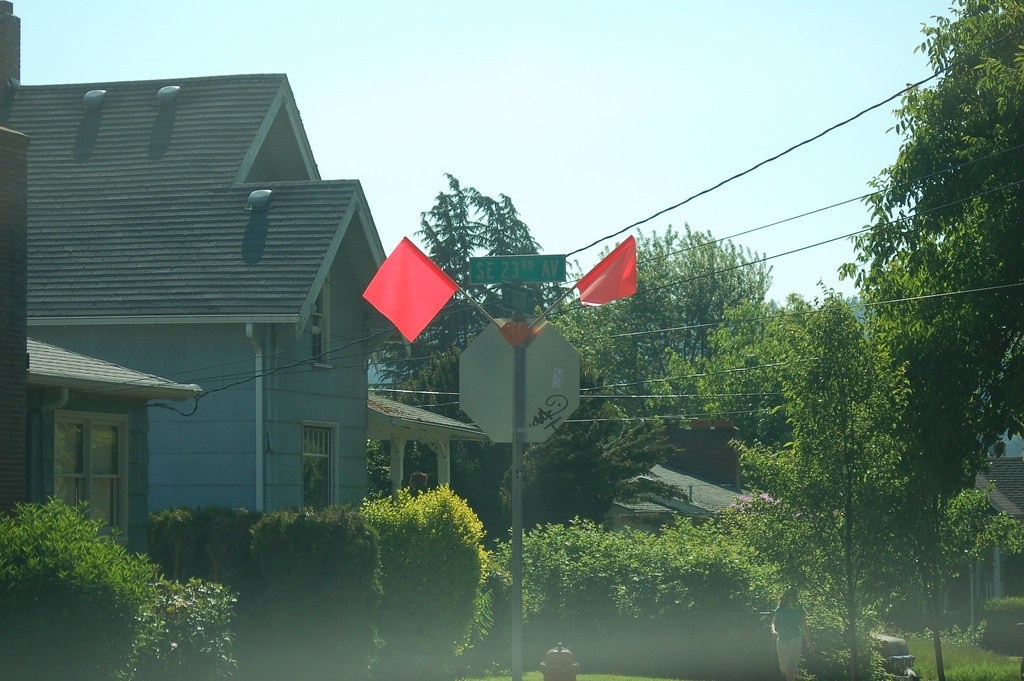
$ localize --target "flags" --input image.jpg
[576,235,637,306]
[362,236,458,343]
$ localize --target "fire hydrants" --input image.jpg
[539,642,580,680]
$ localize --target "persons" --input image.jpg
[410,473,428,498]
[772,585,816,681]
